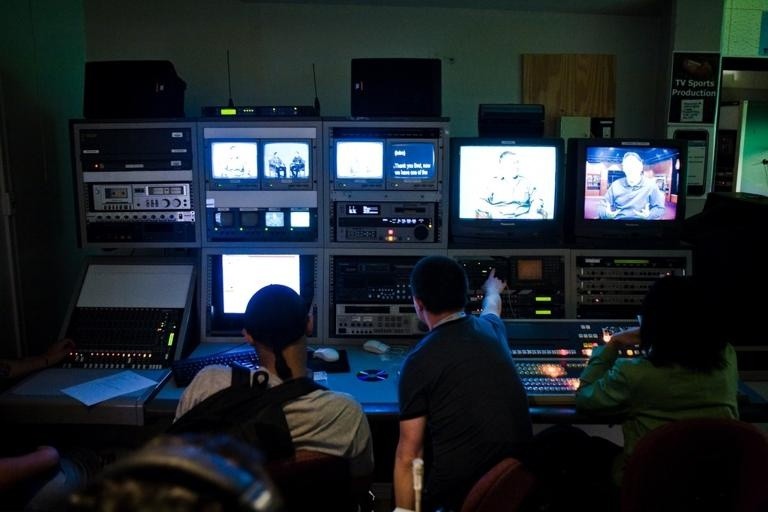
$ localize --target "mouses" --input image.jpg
[313,347,339,362]
[363,340,391,354]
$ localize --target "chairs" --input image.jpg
[458,453,553,512]
[271,448,373,512]
[0,444,62,510]
[619,412,768,512]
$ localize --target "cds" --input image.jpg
[356,369,389,382]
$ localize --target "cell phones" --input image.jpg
[672,128,709,196]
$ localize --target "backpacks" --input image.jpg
[168,362,329,459]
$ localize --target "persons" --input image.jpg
[602,151,667,220]
[44,429,282,510]
[575,275,739,493]
[392,254,533,512]
[0,337,102,512]
[170,284,375,478]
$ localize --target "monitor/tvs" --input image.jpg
[351,57,442,118]
[83,60,186,119]
[386,139,438,190]
[333,138,386,190]
[222,254,300,313]
[260,139,312,190]
[207,139,260,190]
[565,138,688,250]
[448,137,565,249]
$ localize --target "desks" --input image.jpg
[165,340,709,419]
[3,366,169,429]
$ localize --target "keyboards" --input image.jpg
[171,350,267,388]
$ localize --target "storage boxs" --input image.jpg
[676,0,766,59]
